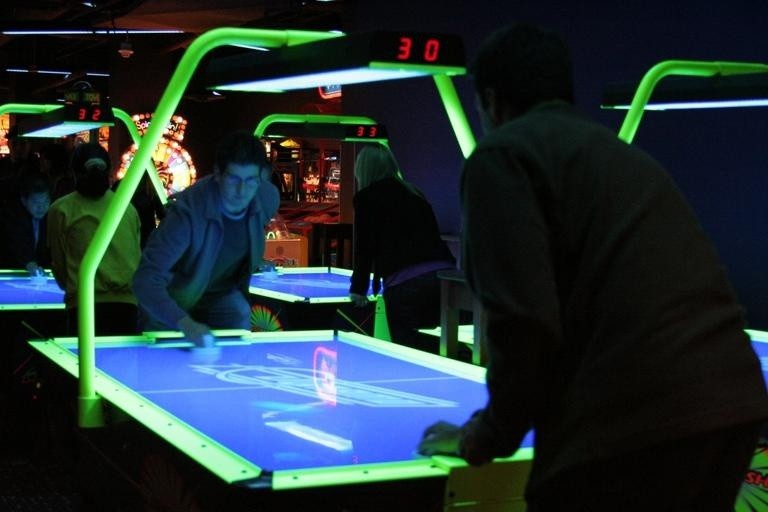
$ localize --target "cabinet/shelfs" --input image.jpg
[259,136,366,267]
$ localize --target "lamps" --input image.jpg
[118,33,134,58]
[17,104,115,139]
[600,72,768,110]
[205,34,466,95]
[263,124,389,143]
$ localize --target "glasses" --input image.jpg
[221,170,262,187]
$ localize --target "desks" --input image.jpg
[0,269,66,337]
[249,267,383,330]
[27,331,534,511]
[437,271,488,366]
[734,328,768,512]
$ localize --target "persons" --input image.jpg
[418,53,767,512]
[131,132,281,464]
[349,145,457,352]
[2,124,215,401]
